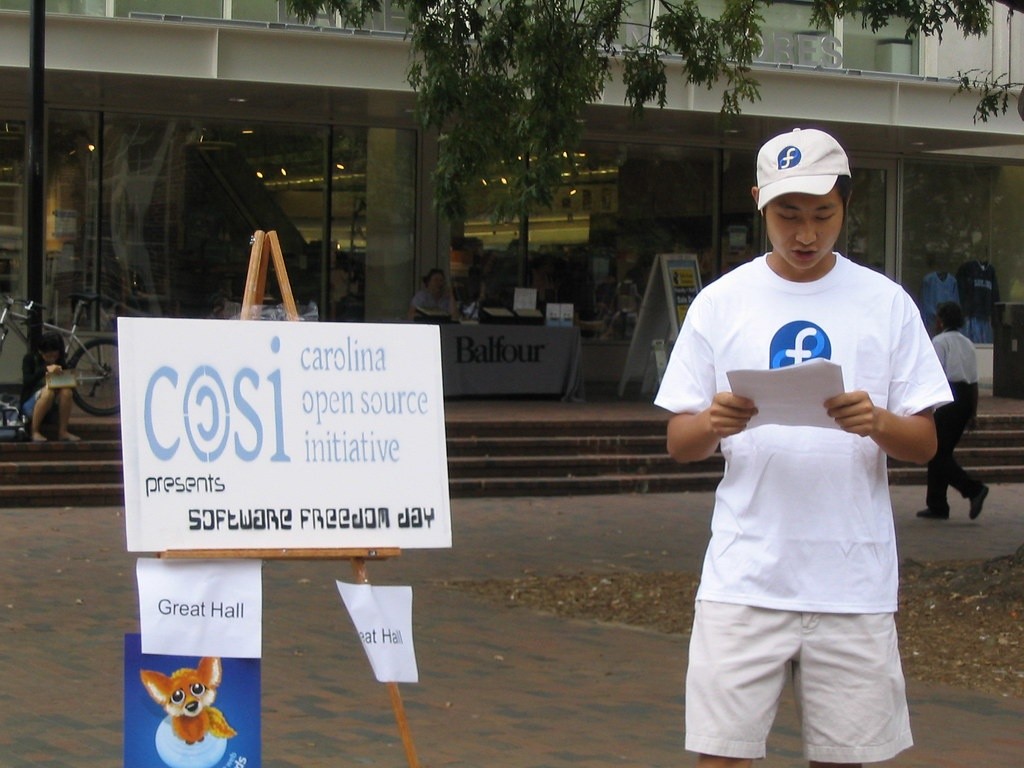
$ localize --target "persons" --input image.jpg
[17,331,81,442]
[654,128,954,768]
[916,300,989,521]
[921,258,1000,343]
[407,269,458,321]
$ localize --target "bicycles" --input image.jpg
[0,292,120,417]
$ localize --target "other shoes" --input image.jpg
[917,506,949,519]
[32,433,47,441]
[59,434,81,441]
[969,485,989,519]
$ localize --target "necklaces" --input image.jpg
[977,259,989,271]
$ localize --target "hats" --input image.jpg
[756,128,852,211]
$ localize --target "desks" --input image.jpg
[439,319,588,404]
